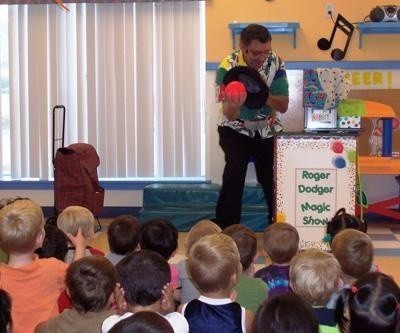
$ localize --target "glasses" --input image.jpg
[248,48,273,59]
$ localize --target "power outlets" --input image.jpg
[324,3,334,19]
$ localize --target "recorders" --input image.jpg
[370,5,400,21]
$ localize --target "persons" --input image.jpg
[1,194,398,333]
[214,23,289,231]
